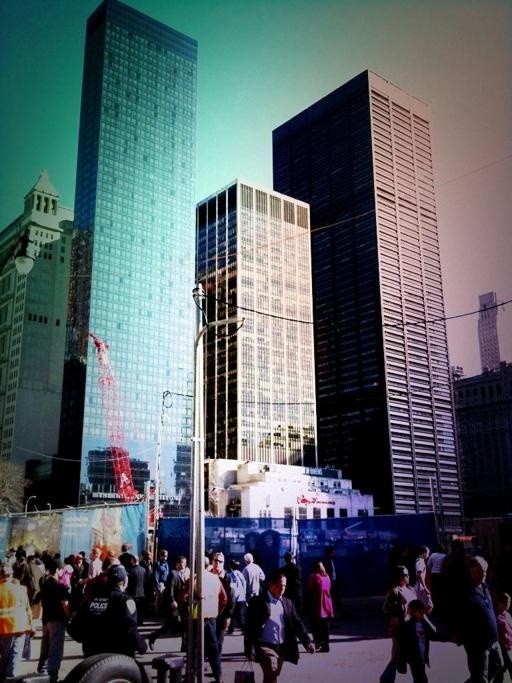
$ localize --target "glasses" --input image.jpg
[213,558,224,564]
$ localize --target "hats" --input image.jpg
[73,554,86,561]
[106,564,130,583]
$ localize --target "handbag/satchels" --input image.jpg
[234,657,255,683]
